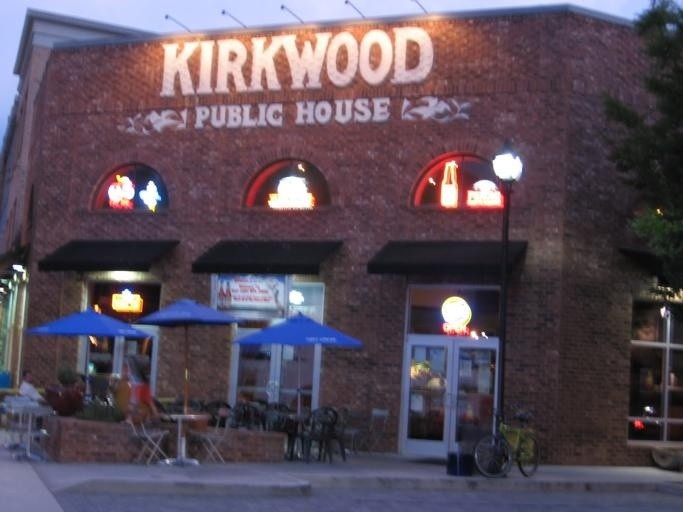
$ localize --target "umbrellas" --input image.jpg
[128,299,240,410]
[22,305,150,402]
[232,309,365,410]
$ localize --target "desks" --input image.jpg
[0,394,58,464]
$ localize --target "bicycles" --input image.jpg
[471,410,542,478]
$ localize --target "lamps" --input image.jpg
[165,0,428,33]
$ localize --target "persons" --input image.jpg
[18,369,50,443]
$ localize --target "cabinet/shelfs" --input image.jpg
[626,291,683,449]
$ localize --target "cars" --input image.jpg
[238,377,313,411]
[628,401,662,442]
[407,387,493,441]
[88,348,151,406]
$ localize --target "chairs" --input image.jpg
[108,380,389,466]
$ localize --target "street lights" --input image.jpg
[484,138,523,469]
